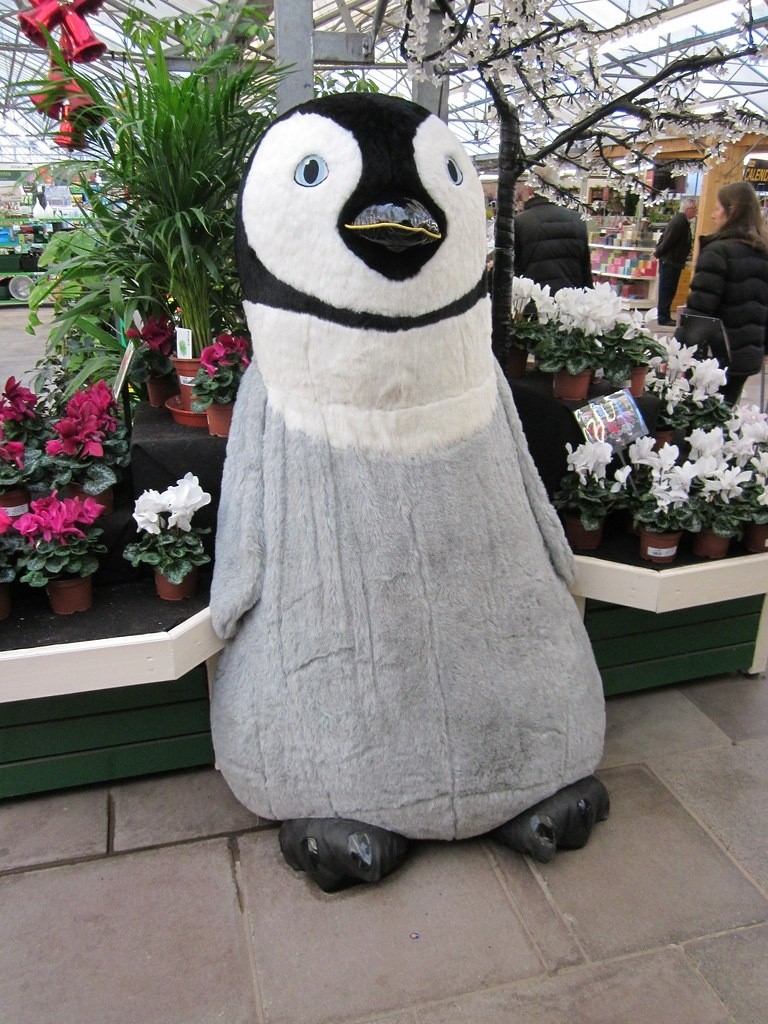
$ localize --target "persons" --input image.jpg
[649,198,697,327]
[514,165,593,298]
[683,181,768,410]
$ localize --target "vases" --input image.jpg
[144,373,176,409]
[51,575,94,614]
[691,524,736,558]
[550,363,592,400]
[745,520,767,552]
[640,526,681,567]
[610,363,646,397]
[202,399,235,436]
[0,585,13,620]
[569,500,605,549]
[1,494,24,507]
[65,484,114,516]
[154,565,195,600]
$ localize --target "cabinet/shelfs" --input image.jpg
[589,214,660,312]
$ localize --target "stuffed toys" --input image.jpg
[209,93,611,894]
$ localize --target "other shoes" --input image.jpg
[663,319,676,326]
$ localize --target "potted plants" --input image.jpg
[132,40,266,427]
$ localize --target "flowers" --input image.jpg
[17,493,108,587]
[0,375,45,493]
[0,507,14,582]
[125,317,172,371]
[46,381,129,494]
[564,413,768,515]
[498,276,728,414]
[190,332,248,413]
[124,473,211,583]
